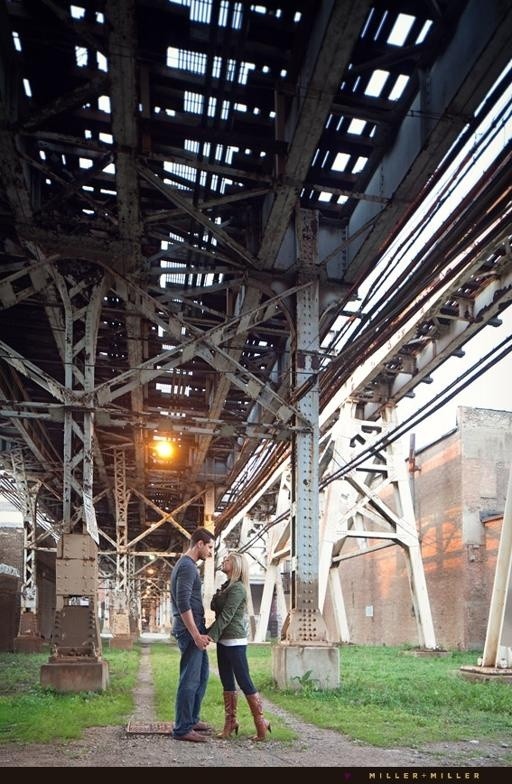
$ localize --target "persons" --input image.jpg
[205,551,273,741]
[169,527,216,742]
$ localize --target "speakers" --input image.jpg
[216,690,239,739]
[246,691,272,741]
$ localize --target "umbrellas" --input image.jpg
[191,721,209,730]
[174,728,207,742]
